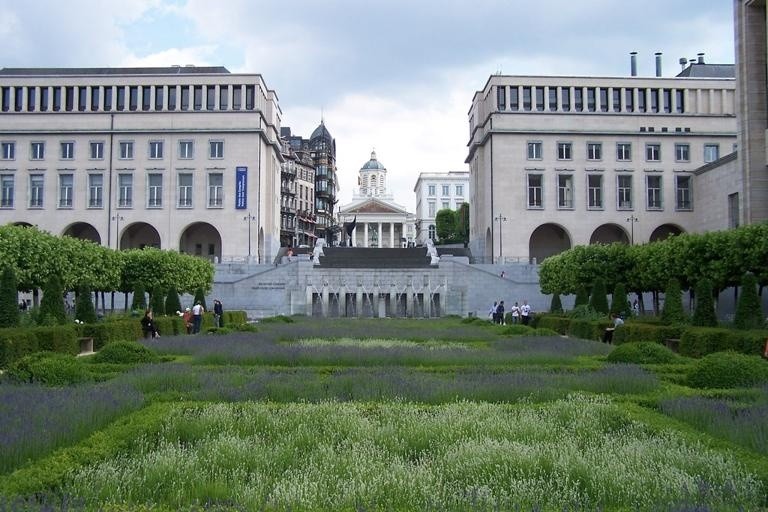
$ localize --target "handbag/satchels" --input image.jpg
[199,307,203,315]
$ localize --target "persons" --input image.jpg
[495,301,505,325]
[212,299,224,328]
[18,299,27,313]
[139,308,162,339]
[63,299,76,315]
[287,248,293,257]
[488,301,498,325]
[500,271,506,276]
[510,300,531,326]
[602,312,625,344]
[174,300,205,336]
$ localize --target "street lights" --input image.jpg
[112,212,124,250]
[495,213,508,256]
[626,214,639,247]
[243,212,256,256]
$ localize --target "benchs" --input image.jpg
[76,336,96,352]
[666,339,680,353]
[141,328,153,339]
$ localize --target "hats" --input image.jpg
[186,308,191,312]
